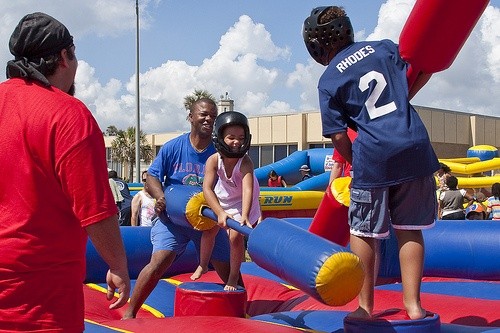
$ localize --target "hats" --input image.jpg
[466,204,486,218]
[299,165,310,171]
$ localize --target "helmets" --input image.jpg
[212,111,251,158]
[302,6,354,66]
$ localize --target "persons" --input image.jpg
[299,165,312,181]
[108,170,158,227]
[303,6,442,321]
[435,162,500,220]
[120,90,260,321]
[190,111,261,292]
[0,12,131,333]
[268,170,287,188]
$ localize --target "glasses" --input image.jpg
[142,179,147,182]
